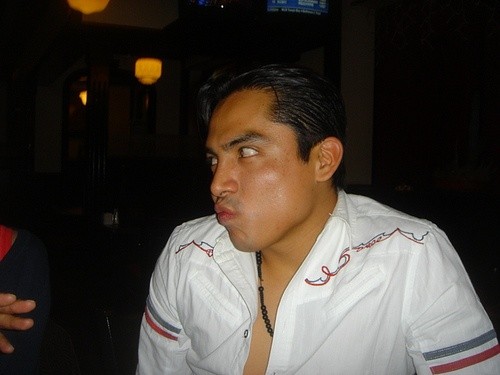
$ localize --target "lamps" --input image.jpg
[66,0,110,14]
[77,85,89,106]
[134,57,163,86]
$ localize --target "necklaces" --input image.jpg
[256,250,274,338]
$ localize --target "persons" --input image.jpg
[0,224,51,375]
[134,60,499,374]
